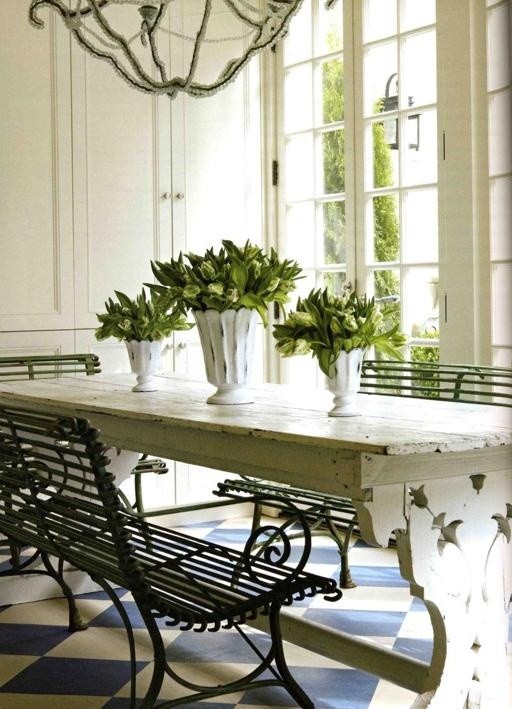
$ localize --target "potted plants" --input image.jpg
[147,237,307,404]
[272,282,408,418]
[94,284,196,392]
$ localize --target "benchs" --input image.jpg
[212,361,512,589]
[0,403,342,709]
[0,354,169,576]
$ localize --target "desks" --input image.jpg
[0,373,512,709]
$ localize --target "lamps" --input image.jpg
[380,73,420,150]
